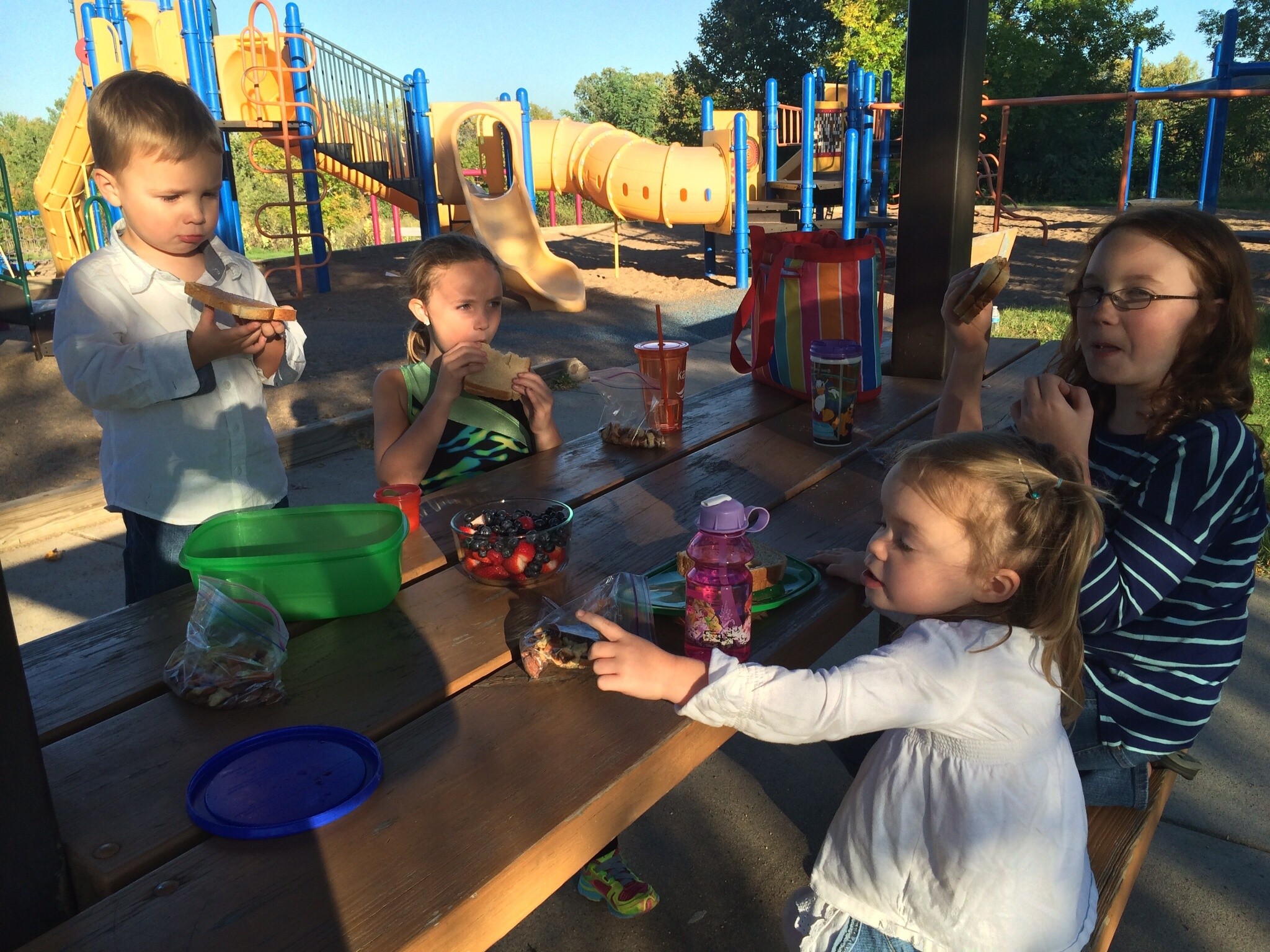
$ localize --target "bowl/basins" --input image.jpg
[178,503,409,621]
[449,497,573,587]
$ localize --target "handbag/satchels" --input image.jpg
[730,225,886,403]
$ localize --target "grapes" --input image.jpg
[461,507,569,576]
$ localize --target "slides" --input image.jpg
[457,168,587,312]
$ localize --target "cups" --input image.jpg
[373,484,423,533]
[810,339,862,447]
[634,340,689,432]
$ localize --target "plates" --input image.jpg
[613,551,821,616]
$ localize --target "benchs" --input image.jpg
[1087,747,1189,952]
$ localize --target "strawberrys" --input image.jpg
[459,527,565,580]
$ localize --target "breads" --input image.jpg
[954,256,1010,324]
[184,281,294,322]
[676,539,786,591]
[464,342,530,401]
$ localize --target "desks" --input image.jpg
[0,330,1062,950]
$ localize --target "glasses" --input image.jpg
[1065,285,1204,311]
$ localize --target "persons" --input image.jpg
[931,203,1270,805]
[574,431,1105,952]
[371,236,563,496]
[52,70,309,609]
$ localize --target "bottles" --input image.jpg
[991,306,1000,333]
[683,494,770,663]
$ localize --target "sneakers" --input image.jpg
[577,843,660,919]
